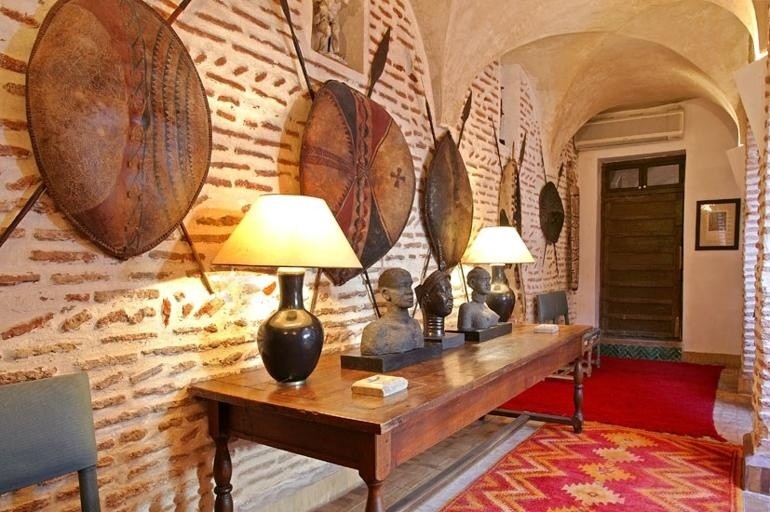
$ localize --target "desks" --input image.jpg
[184,315,596,511]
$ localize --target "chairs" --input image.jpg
[0,369,105,511]
[533,291,609,378]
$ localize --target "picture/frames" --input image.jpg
[696,197,742,251]
[297,0,371,87]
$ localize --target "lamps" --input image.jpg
[209,194,362,390]
[458,226,535,322]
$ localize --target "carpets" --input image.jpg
[496,345,727,442]
[439,407,749,511]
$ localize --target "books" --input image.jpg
[533,323,559,334]
[351,374,409,398]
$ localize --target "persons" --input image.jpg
[360,267,424,356]
[414,270,454,337]
[457,266,501,331]
